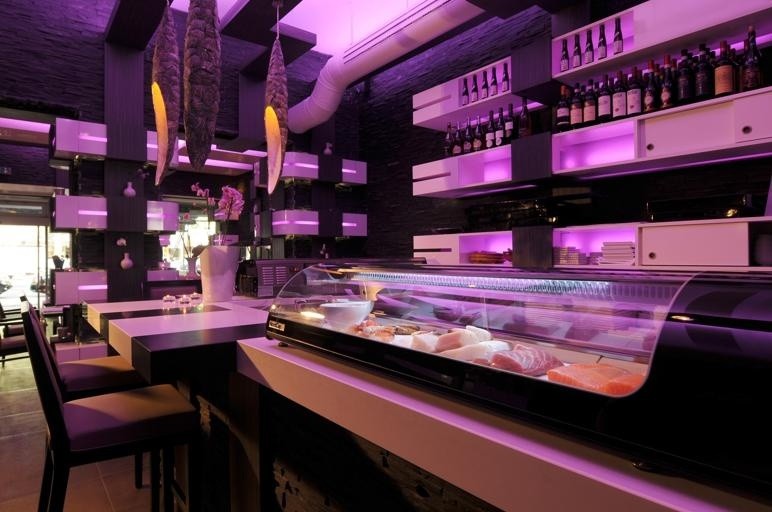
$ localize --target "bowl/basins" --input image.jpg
[318,301,369,332]
[503,252,512,261]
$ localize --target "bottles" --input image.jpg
[461,78,469,106]
[444,97,532,160]
[323,142,331,155]
[553,25,762,134]
[39,275,44,285]
[612,17,623,55]
[491,66,498,95]
[123,182,137,198]
[500,63,509,92]
[481,70,488,99]
[470,74,479,103]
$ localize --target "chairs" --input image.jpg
[20,294,202,511]
[0,293,45,366]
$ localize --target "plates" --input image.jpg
[553,241,635,267]
[468,250,505,265]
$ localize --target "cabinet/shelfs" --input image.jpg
[265,262,772,506]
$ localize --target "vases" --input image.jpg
[123,181,136,197]
[185,257,201,281]
[195,242,241,303]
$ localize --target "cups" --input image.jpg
[57,326,68,337]
[53,188,65,196]
[158,261,171,270]
[163,294,203,313]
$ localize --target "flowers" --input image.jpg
[180,234,208,258]
[180,182,250,245]
[125,160,153,181]
[116,237,130,252]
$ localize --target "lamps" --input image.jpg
[150,0,290,195]
